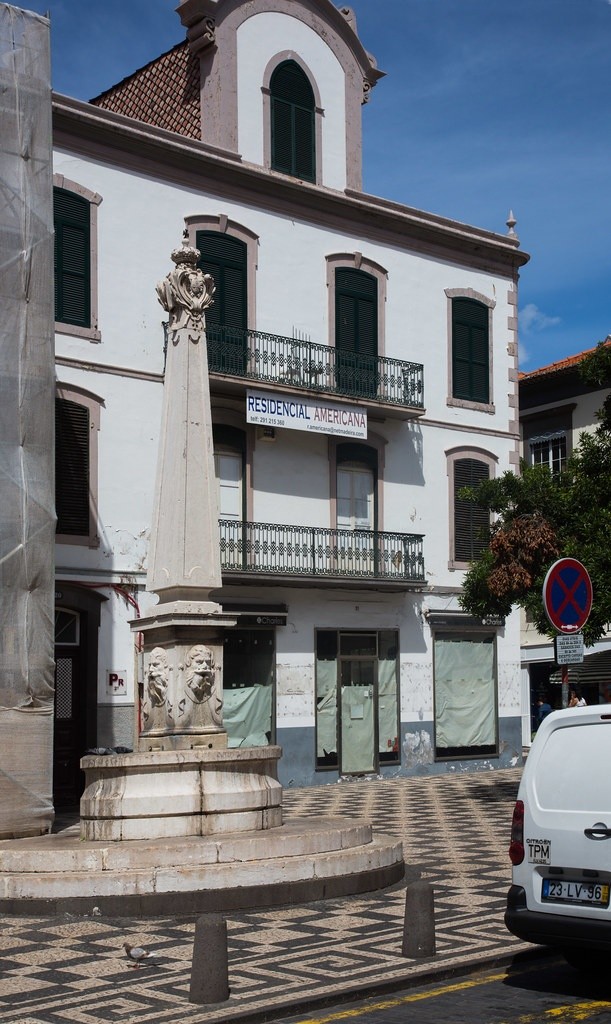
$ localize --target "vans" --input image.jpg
[501,702,611,953]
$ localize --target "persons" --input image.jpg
[535,696,552,732]
[177,644,223,728]
[567,686,586,709]
[143,646,175,729]
